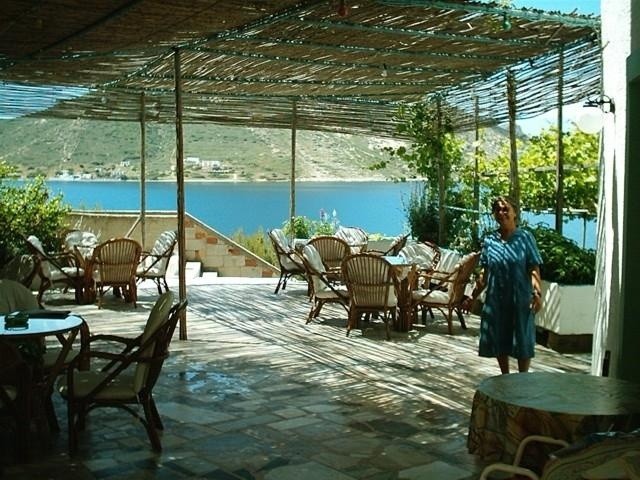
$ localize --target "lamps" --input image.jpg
[576,93,615,136]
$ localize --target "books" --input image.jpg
[28,311,71,319]
[380,255,404,266]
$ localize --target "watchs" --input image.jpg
[468,295,475,302]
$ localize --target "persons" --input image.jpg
[460,195,544,375]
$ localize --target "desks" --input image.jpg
[467,372,640,464]
[1,313,83,454]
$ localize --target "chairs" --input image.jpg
[268,228,481,342]
[61,291,187,467]
[21,310,91,428]
[23,228,176,310]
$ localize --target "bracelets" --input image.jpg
[533,293,541,299]
